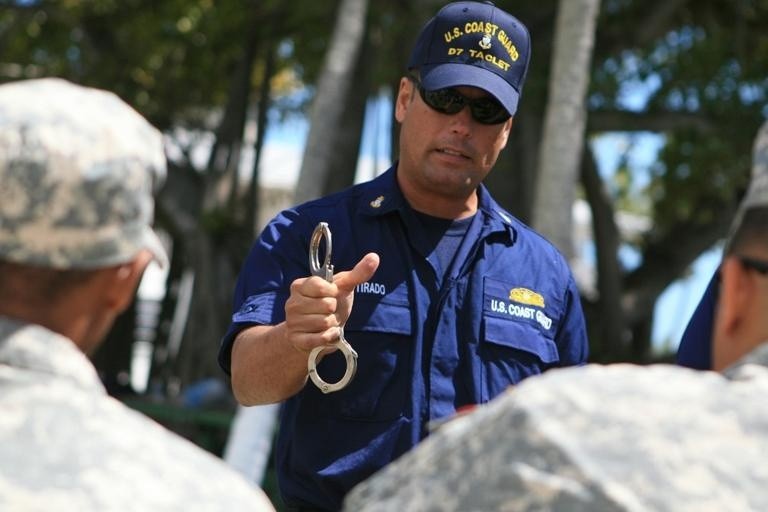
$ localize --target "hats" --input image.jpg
[1,76,169,276]
[723,120,768,256]
[408,0,532,116]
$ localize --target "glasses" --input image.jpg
[409,75,512,124]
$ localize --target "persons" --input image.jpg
[0,74,276,512]
[217,0,589,512]
[342,116,768,512]
